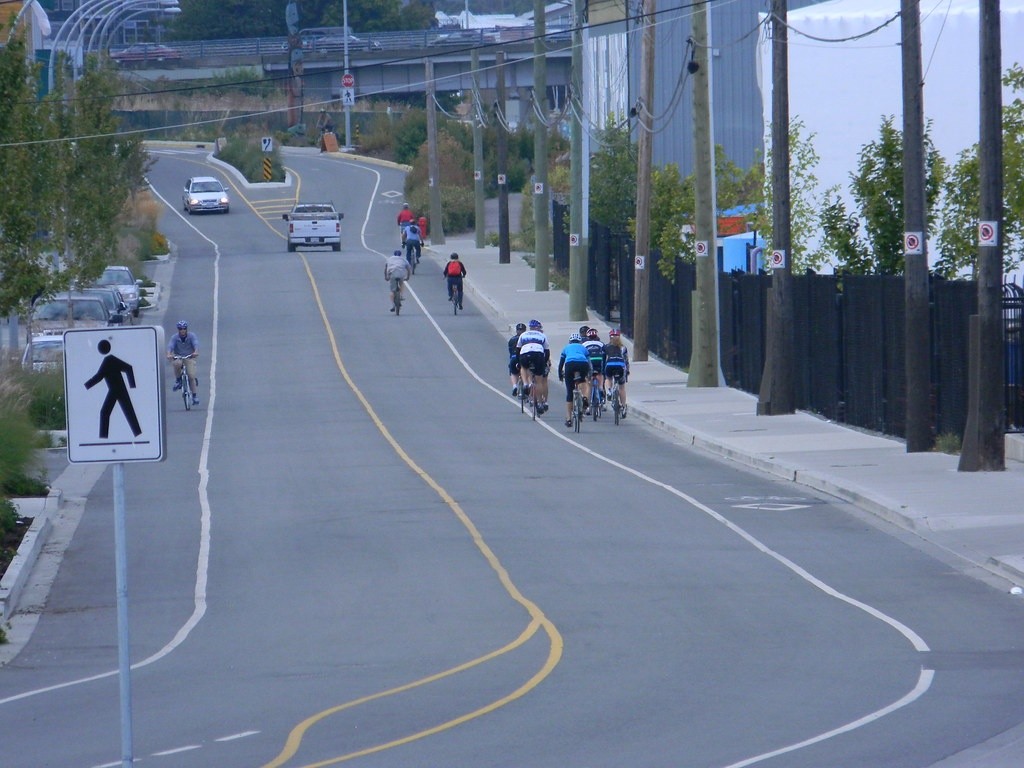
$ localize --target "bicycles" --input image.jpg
[560,373,587,434]
[404,243,420,275]
[170,352,199,410]
[394,277,405,315]
[609,369,624,425]
[591,367,605,422]
[513,364,543,420]
[443,274,459,316]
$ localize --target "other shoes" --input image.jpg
[175,381,182,388]
[193,395,200,404]
[544,402,549,411]
[599,390,606,399]
[523,384,529,396]
[565,419,572,427]
[536,401,544,414]
[416,258,419,263]
[448,296,453,301]
[622,405,626,418]
[582,397,589,409]
[512,387,517,397]
[391,306,395,312]
[607,391,612,401]
[459,304,463,310]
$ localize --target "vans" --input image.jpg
[282,26,354,54]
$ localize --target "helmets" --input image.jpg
[586,328,598,337]
[529,319,541,328]
[403,203,409,209]
[176,321,188,329]
[516,323,526,330]
[568,333,582,342]
[580,325,590,335]
[409,219,415,225]
[394,249,401,256]
[609,329,620,336]
[451,252,458,259]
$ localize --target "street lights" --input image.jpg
[42,1,182,136]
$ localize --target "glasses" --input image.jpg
[179,328,186,330]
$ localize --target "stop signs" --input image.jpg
[342,75,355,89]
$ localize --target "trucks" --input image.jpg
[281,201,345,253]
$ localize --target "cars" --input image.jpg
[428,29,496,47]
[84,267,144,318]
[112,42,184,68]
[20,266,132,374]
[183,177,231,214]
[313,34,384,53]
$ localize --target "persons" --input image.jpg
[167,322,200,404]
[384,250,411,312]
[600,330,630,418]
[508,320,551,415]
[558,334,593,427]
[444,253,466,309]
[579,326,606,415]
[397,203,415,241]
[401,219,424,263]
[317,109,333,133]
